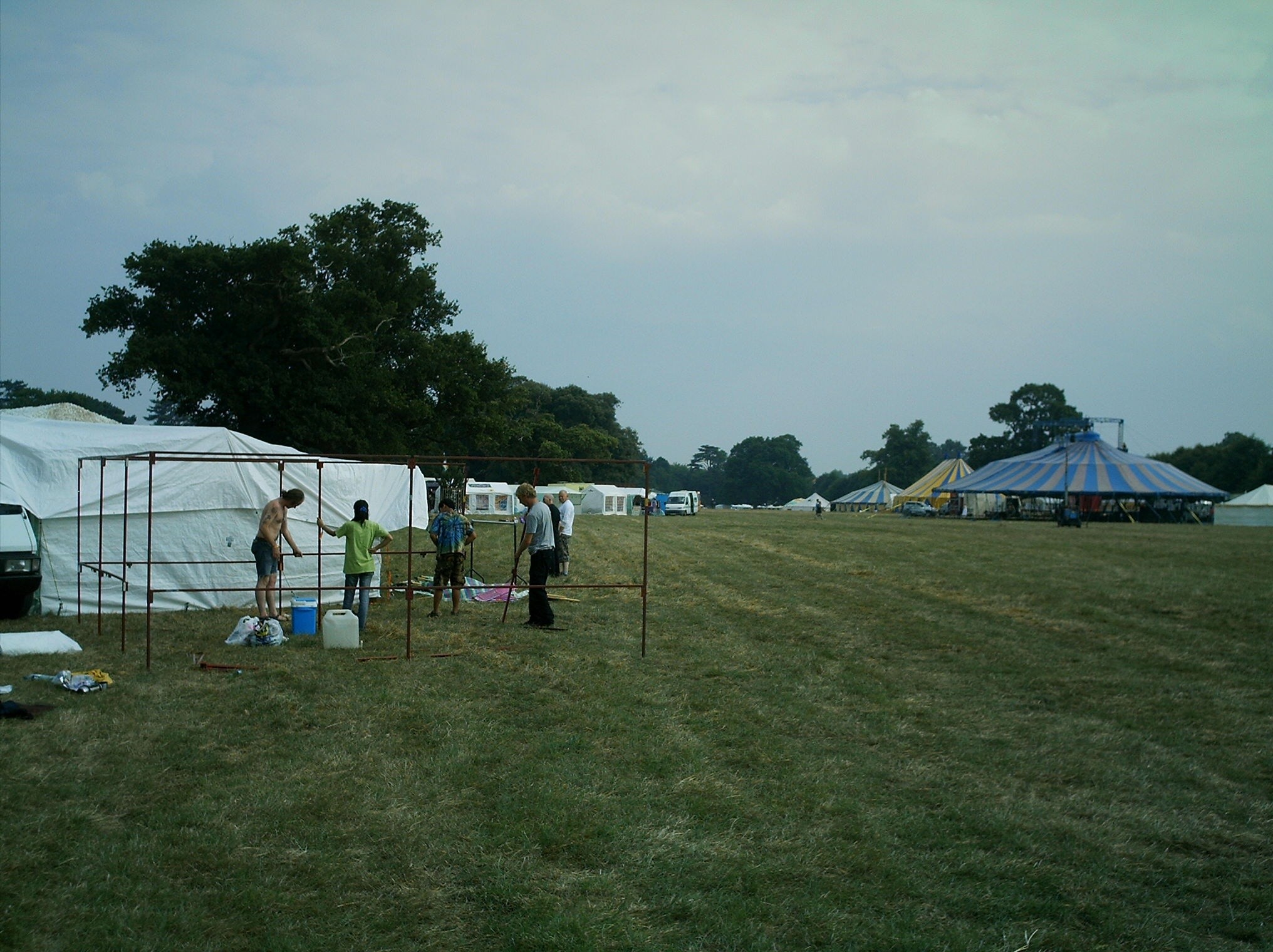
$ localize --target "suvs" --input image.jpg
[903,501,938,518]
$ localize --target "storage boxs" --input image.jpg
[290,598,317,635]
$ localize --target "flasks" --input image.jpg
[78,683,103,694]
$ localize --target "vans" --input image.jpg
[0,500,42,621]
[664,490,698,517]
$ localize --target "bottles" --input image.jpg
[23,674,55,682]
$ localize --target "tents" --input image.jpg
[425,478,672,518]
[1213,483,1273,528]
[893,457,1022,516]
[783,492,831,512]
[832,480,906,512]
[932,431,1232,523]
[0,401,429,613]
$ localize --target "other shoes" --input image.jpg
[560,572,569,576]
[450,611,459,616]
[427,611,443,617]
[551,572,561,577]
[524,620,555,627]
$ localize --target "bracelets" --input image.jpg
[322,525,326,529]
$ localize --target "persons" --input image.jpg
[317,500,393,635]
[542,494,561,577]
[514,483,555,629]
[251,488,304,621]
[427,499,477,618]
[813,499,824,520]
[557,489,574,576]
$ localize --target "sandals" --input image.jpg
[269,615,289,621]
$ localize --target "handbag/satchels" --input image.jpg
[226,615,289,645]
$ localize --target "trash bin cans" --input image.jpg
[290,596,317,636]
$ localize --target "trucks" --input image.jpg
[938,497,963,517]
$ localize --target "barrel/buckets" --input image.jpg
[320,609,360,651]
